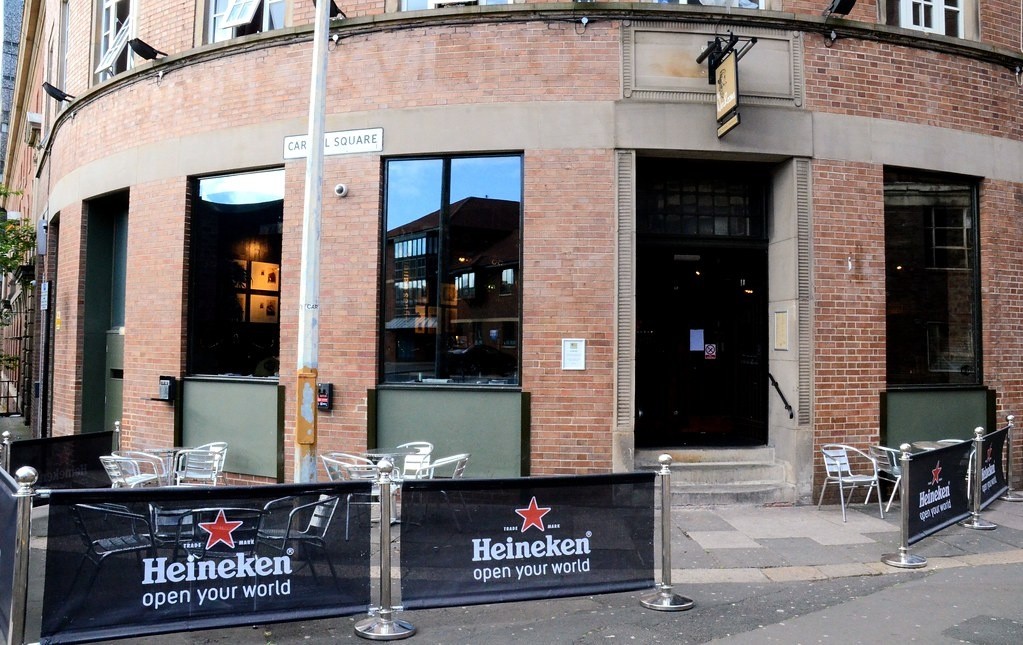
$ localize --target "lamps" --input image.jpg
[823,0,857,18]
[42,81,75,103]
[312,0,347,18]
[126,38,168,61]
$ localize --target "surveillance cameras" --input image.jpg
[335,183,347,196]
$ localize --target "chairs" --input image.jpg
[63,502,197,607]
[242,489,347,600]
[165,506,266,617]
[98,442,229,488]
[320,441,474,542]
[864,444,912,513]
[817,442,884,522]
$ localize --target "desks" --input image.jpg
[912,440,961,451]
[142,447,194,488]
[157,508,259,558]
[359,447,420,480]
